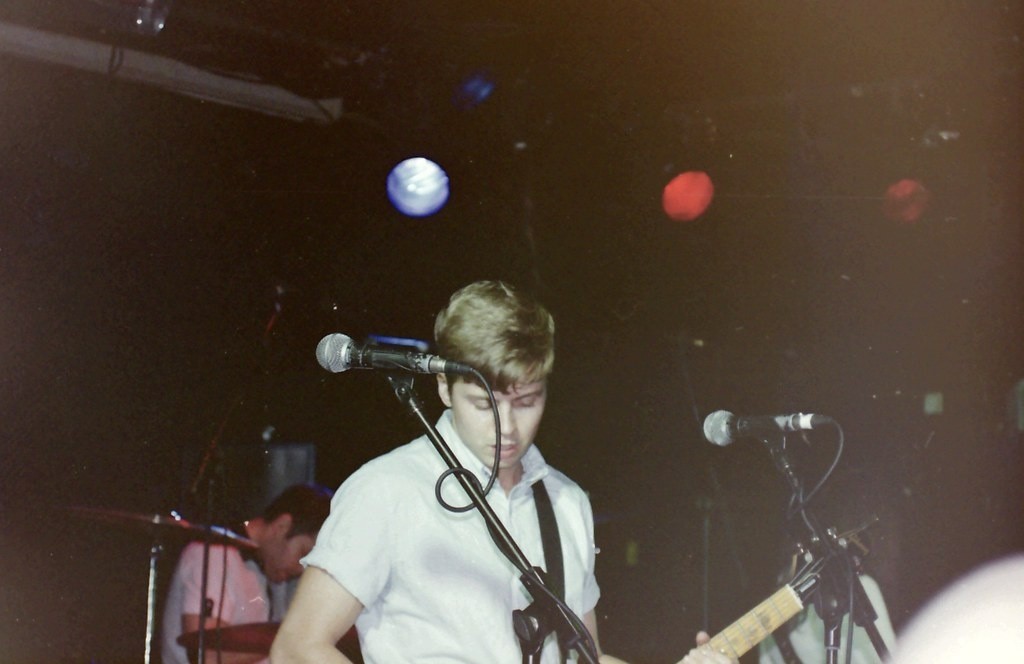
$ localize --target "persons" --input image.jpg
[161,484,333,664]
[757,544,896,664]
[267,283,738,664]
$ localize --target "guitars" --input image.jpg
[678,515,887,663]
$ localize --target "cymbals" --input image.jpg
[59,504,258,552]
[177,619,360,656]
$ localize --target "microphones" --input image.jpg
[316,332,475,374]
[704,410,833,446]
